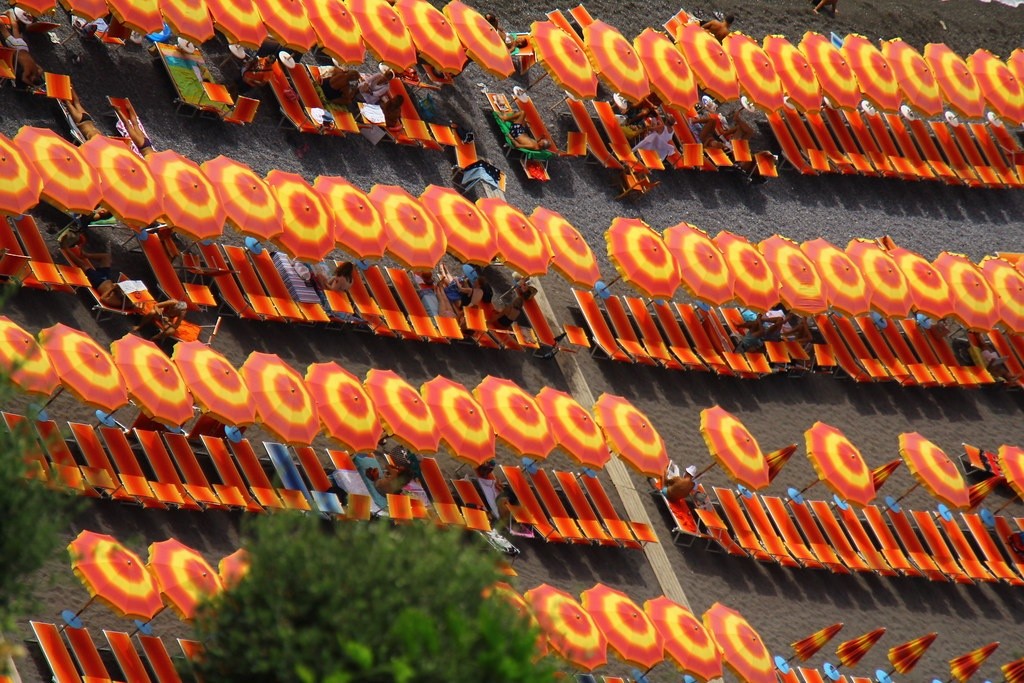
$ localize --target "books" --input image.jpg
[668,459,680,478]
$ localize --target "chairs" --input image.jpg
[1,3,1024,683]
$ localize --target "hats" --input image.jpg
[686,465,697,477]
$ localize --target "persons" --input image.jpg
[495,477,521,526]
[431,257,524,334]
[701,13,735,42]
[153,297,187,343]
[732,303,816,368]
[478,12,553,150]
[2,10,157,158]
[623,88,758,168]
[973,330,1015,383]
[244,49,404,136]
[367,442,425,497]
[813,0,840,15]
[660,459,696,500]
[314,259,353,292]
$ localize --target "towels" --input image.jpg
[493,110,557,162]
[477,477,514,526]
[155,40,230,115]
[738,305,796,340]
[327,449,398,518]
[632,132,675,164]
[415,289,456,327]
[271,250,321,304]
[462,160,500,189]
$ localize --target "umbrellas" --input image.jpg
[0,125,1024,336]
[0,312,1024,504]
[16,0,1024,124]
[65,532,1024,683]
